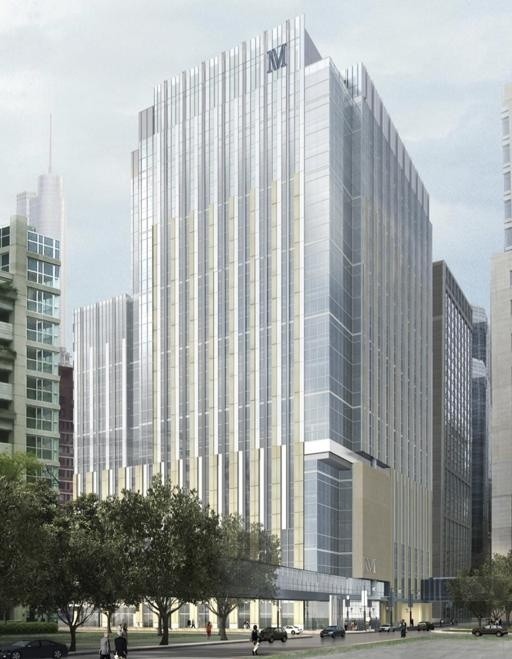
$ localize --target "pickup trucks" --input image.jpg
[378,624,395,632]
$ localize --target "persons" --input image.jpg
[122,622,128,645]
[98,633,111,659]
[250,624,261,656]
[191,619,195,628]
[113,630,129,658]
[205,621,213,640]
[399,619,408,638]
[187,618,191,628]
[117,623,123,638]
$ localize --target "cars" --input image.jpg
[320,626,345,639]
[472,624,508,637]
[283,625,303,635]
[0,638,69,659]
[258,626,288,644]
[417,622,435,632]
